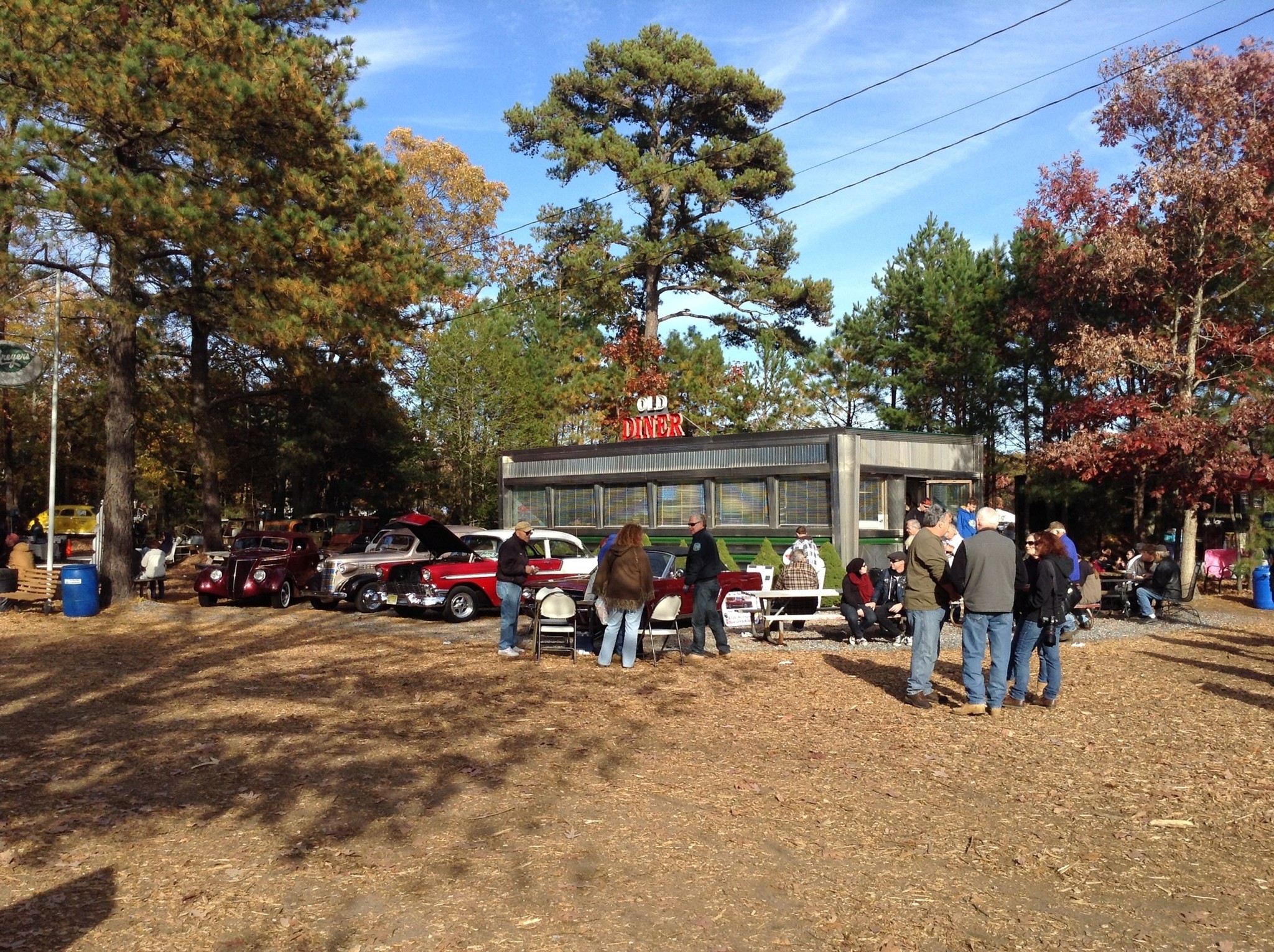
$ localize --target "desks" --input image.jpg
[36,563,69,569]
[30,543,60,562]
[1093,579,1150,616]
[176,544,192,556]
[68,555,92,564]
[741,589,843,645]
[576,600,604,653]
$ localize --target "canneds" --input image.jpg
[1132,570,1136,579]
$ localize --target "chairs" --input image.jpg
[533,588,578,664]
[1204,565,1236,595]
[634,593,683,667]
[1154,562,1201,624]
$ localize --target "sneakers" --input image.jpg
[497,647,519,656]
[512,645,525,652]
[905,691,1056,716]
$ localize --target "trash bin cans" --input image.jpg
[61,563,101,615]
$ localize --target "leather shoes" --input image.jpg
[1079,621,1089,628]
[1139,616,1158,624]
[1059,628,1078,641]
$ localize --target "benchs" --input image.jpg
[735,607,843,634]
[0,568,61,616]
[133,577,167,598]
[1096,595,1132,618]
[1072,603,1100,630]
[761,614,901,643]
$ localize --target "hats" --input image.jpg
[10,533,19,542]
[1148,545,1167,551]
[887,551,908,562]
[515,521,534,532]
[846,558,864,574]
[921,498,932,507]
[1044,521,1066,532]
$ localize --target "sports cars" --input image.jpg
[520,545,765,633]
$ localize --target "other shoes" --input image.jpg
[849,634,913,647]
[716,653,732,659]
[685,653,704,661]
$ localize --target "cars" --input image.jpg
[194,514,601,623]
[24,503,98,534]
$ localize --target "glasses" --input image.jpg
[1034,541,1039,546]
[1025,541,1034,545]
[688,521,701,526]
[862,565,866,568]
[518,529,533,535]
[890,560,901,563]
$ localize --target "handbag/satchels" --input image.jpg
[1060,582,1082,616]
[595,595,609,625]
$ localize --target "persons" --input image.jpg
[592,523,655,668]
[0,517,173,599]
[949,507,1029,716]
[902,496,978,568]
[1001,521,1182,709]
[841,558,877,646]
[496,522,540,656]
[682,513,732,659]
[865,551,915,646]
[792,526,819,568]
[771,550,819,632]
[903,504,961,708]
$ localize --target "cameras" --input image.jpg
[1040,615,1060,647]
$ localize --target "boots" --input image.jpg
[1006,680,1012,695]
[1028,681,1047,700]
